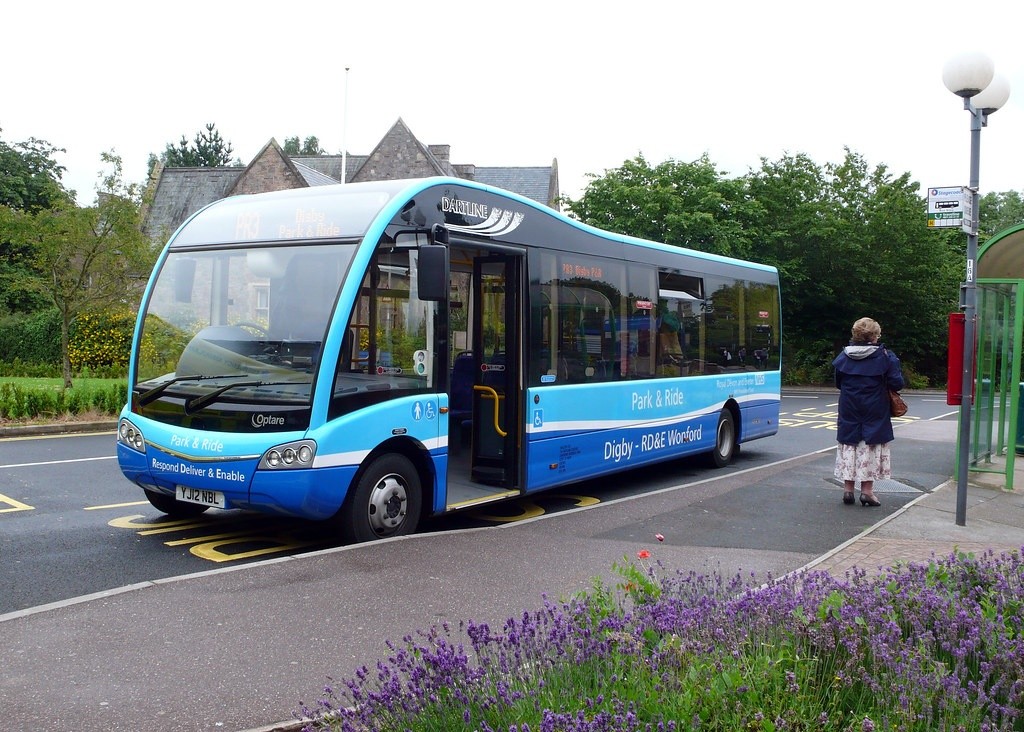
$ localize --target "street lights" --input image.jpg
[939,45,1011,528]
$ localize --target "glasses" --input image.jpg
[875,335,881,338]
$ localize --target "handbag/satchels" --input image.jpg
[660,331,684,361]
[888,388,908,417]
[727,352,732,360]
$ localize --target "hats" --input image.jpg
[743,348,745,351]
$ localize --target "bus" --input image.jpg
[116,176,782,543]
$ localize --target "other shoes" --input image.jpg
[743,364,745,367]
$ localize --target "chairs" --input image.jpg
[451,350,720,431]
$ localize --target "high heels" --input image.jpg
[843,491,855,505]
[860,493,881,507]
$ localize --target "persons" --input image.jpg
[833,317,908,508]
